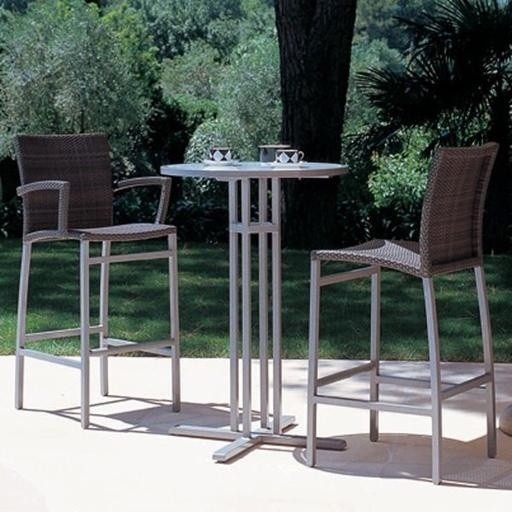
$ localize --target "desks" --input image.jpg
[158,161,349,462]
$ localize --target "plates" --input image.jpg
[266,163,308,169]
[204,161,242,166]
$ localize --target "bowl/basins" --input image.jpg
[257,144,291,162]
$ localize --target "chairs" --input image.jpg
[302,140,501,484]
[9,130,182,431]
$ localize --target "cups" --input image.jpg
[274,149,304,163]
[208,146,240,161]
